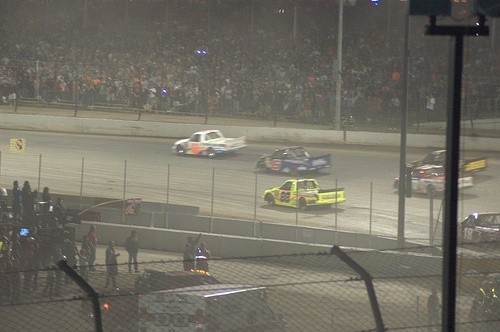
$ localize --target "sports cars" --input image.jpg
[394,165,474,198]
[263,179,347,211]
[409,150,489,173]
[255,145,332,175]
[174,129,248,159]
[459,212,500,243]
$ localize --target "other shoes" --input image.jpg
[133,269,141,272]
[126,269,131,273]
[113,287,119,291]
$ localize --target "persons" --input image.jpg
[125,229,141,273]
[428,287,441,332]
[467,273,499,332]
[184,233,211,272]
[104,241,121,289]
[0,181,98,303]
[0,0,500,130]
